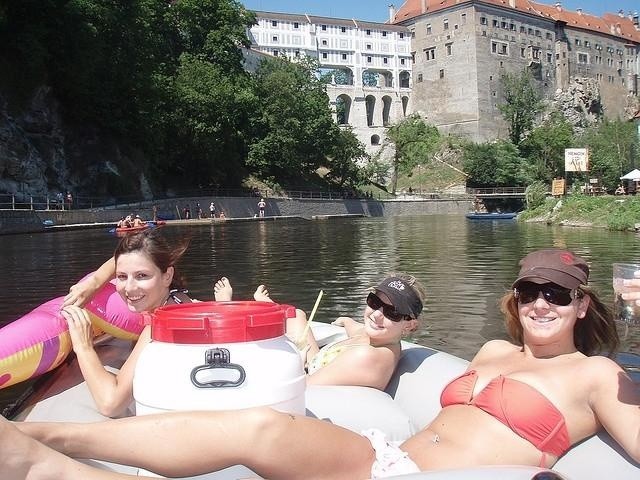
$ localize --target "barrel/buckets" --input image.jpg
[131,298,308,480]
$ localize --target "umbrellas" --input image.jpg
[620,169,640,190]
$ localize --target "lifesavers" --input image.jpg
[0,273,152,388]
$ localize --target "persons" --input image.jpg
[183,204,192,218]
[61,226,207,418]
[1,248,640,477]
[368,190,373,200]
[408,186,412,195]
[210,202,216,218]
[196,203,203,219]
[118,213,146,229]
[258,199,266,217]
[152,204,159,221]
[213,273,426,392]
[615,184,622,195]
[54,190,74,210]
[220,211,225,218]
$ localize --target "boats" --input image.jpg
[0,286,640,480]
[106,221,165,235]
[467,212,518,220]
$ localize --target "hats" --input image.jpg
[511,250,589,290]
[373,277,423,319]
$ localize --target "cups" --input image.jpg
[608,261,640,327]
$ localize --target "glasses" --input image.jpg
[366,292,407,322]
[514,281,580,306]
